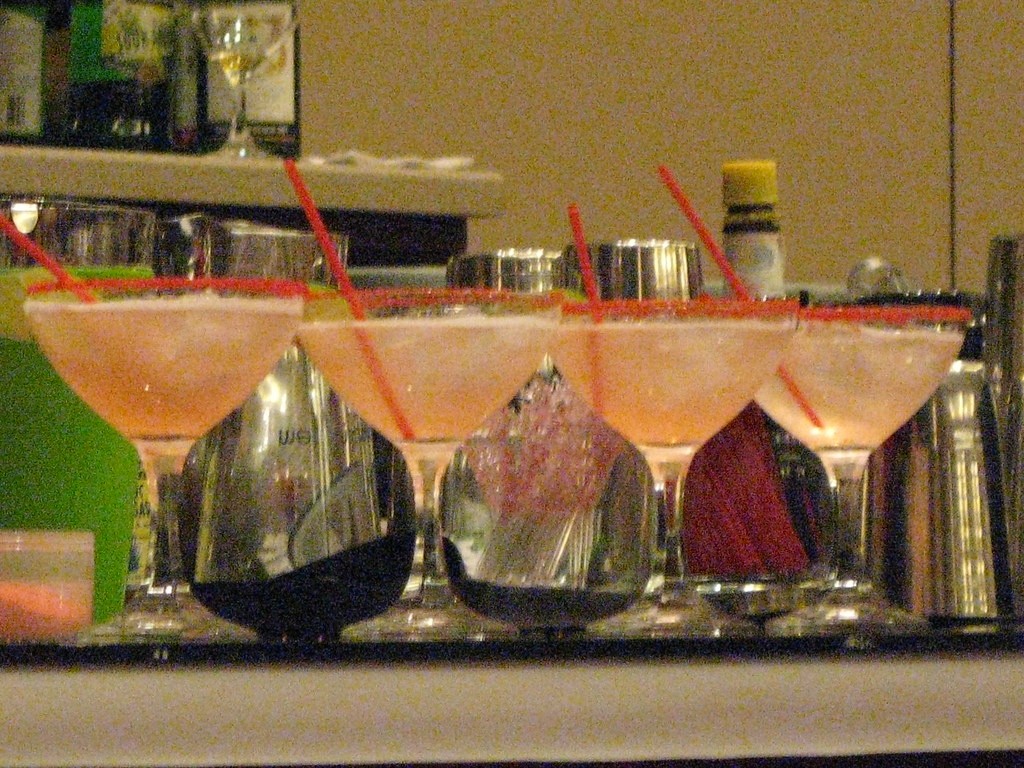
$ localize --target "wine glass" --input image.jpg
[296,290,561,639]
[753,306,972,636]
[190,1,303,162]
[548,301,798,636]
[24,282,304,636]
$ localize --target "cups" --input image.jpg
[0,195,156,266]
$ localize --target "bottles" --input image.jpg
[1,0,308,161]
[2,529,95,643]
[722,161,804,546]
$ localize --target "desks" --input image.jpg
[0,145,504,289]
